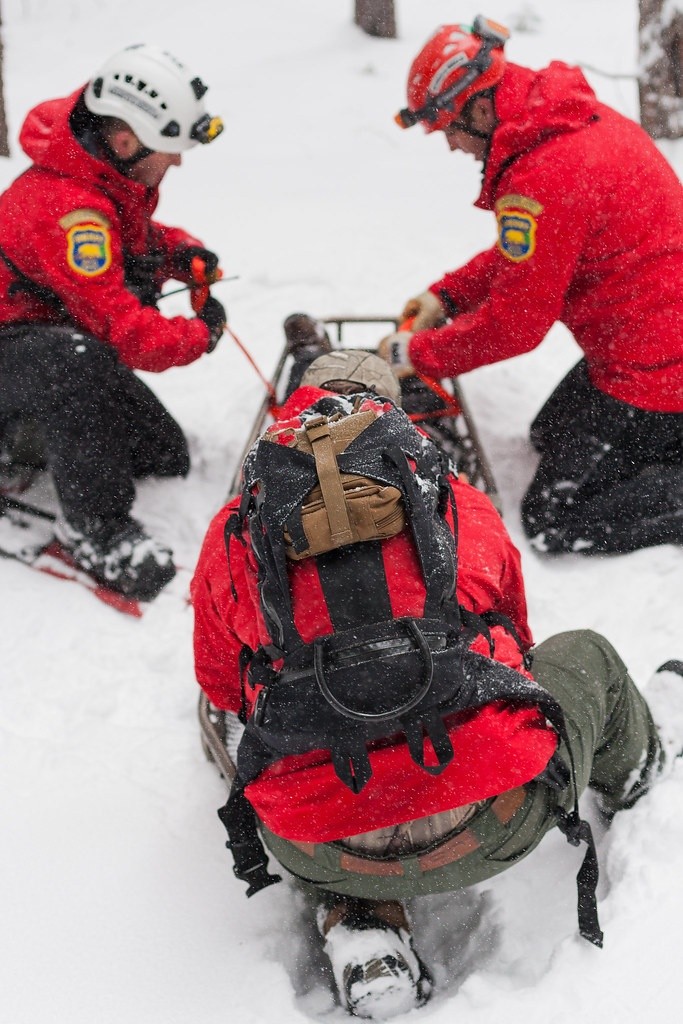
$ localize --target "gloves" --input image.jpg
[179,246,218,275]
[197,296,226,353]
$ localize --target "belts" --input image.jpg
[288,786,527,876]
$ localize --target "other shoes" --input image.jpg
[0,414,49,498]
[320,901,436,1020]
[52,511,176,602]
[593,659,683,815]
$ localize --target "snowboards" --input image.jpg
[0,491,199,621]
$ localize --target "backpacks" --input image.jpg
[224,393,458,559]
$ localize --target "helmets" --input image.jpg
[84,43,224,154]
[299,350,402,408]
[395,15,510,134]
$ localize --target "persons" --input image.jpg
[376,15,683,559]
[0,42,229,605]
[189,347,683,1020]
